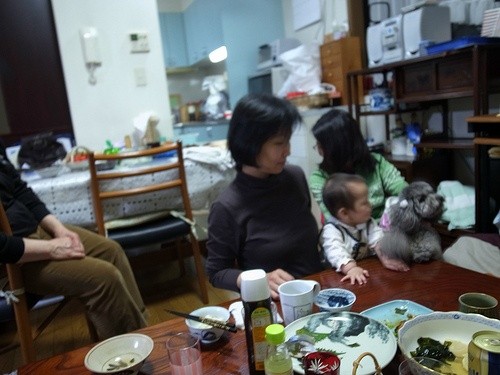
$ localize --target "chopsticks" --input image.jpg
[166,309,237,334]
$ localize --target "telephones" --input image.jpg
[80,26,101,65]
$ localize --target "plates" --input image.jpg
[359,299,433,337]
[283,311,397,375]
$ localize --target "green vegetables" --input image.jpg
[409,336,456,371]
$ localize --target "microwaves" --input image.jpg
[255,37,301,70]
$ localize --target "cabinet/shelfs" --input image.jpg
[321,35,500,235]
[159,0,284,142]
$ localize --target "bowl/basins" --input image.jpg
[84,333,154,375]
[397,311,500,375]
[184,306,230,343]
[38,165,64,178]
[313,288,356,313]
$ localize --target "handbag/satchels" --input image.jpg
[18,138,66,171]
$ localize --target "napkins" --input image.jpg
[229,300,283,327]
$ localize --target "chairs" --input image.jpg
[1,135,209,368]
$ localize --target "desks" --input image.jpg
[25,140,236,240]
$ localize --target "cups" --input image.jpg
[458,293,498,318]
[277,279,321,326]
[166,332,203,375]
[240,301,277,326]
[303,351,341,375]
[399,357,453,375]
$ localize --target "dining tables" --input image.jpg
[18,257,500,374]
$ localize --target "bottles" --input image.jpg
[241,268,274,375]
[263,324,293,375]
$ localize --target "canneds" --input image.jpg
[467,330,500,375]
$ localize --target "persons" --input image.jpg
[0,146,148,338]
[318,173,384,284]
[206,93,319,300]
[309,109,409,225]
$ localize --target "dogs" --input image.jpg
[377,180,446,264]
